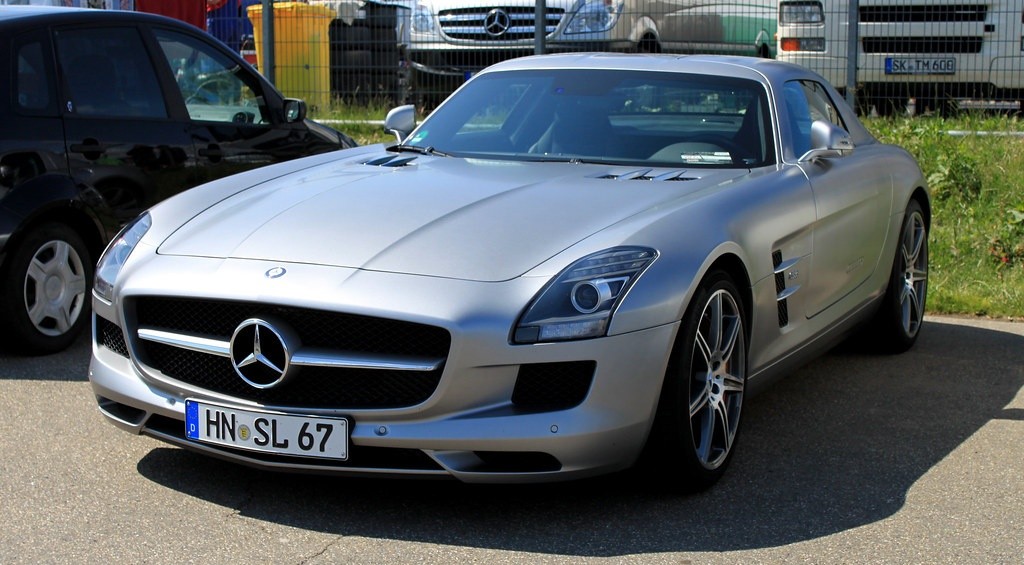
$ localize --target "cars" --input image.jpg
[88,52,932,493]
[0,6,359,358]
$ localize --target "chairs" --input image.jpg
[34,55,166,118]
[528,98,765,161]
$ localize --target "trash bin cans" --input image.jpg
[246,0,337,121]
